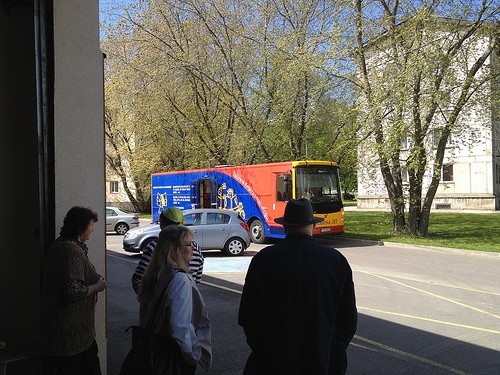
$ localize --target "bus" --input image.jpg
[150,158,347,246]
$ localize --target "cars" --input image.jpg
[122,208,252,259]
[105,207,140,235]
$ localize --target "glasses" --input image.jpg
[181,241,192,247]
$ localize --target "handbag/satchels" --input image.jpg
[118,325,196,375]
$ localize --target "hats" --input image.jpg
[274,198,324,227]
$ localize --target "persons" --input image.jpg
[32,207,108,375]
[238,198,357,375]
[139,225,213,375]
[132,208,204,302]
